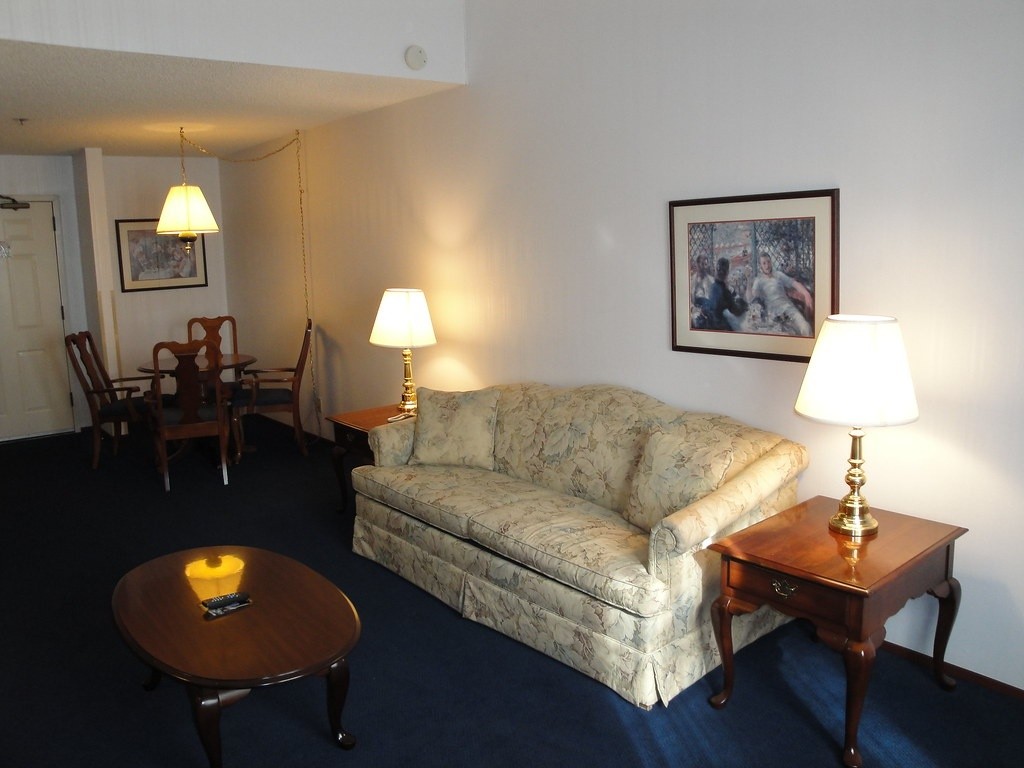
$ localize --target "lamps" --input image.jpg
[185,547,245,610]
[794,314,921,538]
[155,127,220,256]
[837,541,881,590]
[369,288,439,411]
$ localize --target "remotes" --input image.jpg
[202,592,249,610]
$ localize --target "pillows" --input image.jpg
[407,386,502,471]
[622,429,735,532]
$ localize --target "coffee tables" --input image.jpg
[111,545,361,768]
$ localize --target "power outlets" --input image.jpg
[312,398,323,412]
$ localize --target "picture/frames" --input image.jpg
[115,218,209,293]
[670,187,840,363]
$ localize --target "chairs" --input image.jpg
[231,319,313,464]
[187,316,243,406]
[65,331,175,470]
[146,339,230,492]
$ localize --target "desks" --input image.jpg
[704,495,969,768]
[326,404,406,516]
[138,352,258,461]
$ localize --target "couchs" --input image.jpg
[351,383,811,710]
